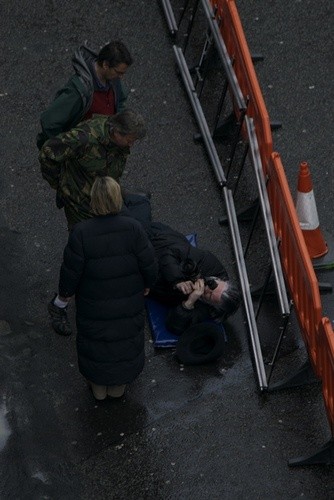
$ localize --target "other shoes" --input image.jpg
[47,292,72,336]
[89,384,125,403]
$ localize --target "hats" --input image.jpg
[175,322,225,366]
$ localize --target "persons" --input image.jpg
[141,221,242,336]
[38,109,146,235]
[47,177,157,404]
[37,39,135,151]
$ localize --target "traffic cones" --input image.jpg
[294,160,334,271]
